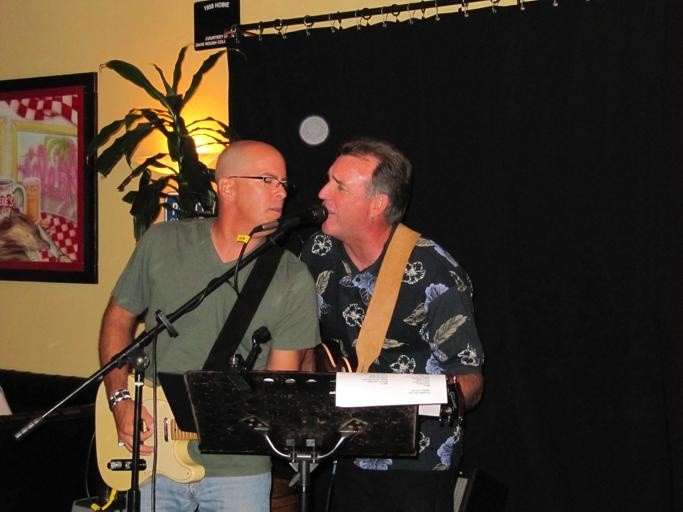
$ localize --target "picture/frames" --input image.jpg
[0,72,100,283]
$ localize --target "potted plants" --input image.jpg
[96,45,302,510]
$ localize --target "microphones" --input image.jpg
[249,202,329,235]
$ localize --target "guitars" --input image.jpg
[94,374,206,490]
[313,342,466,427]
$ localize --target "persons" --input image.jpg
[99,140,322,512]
[297,138,486,512]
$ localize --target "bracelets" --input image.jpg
[109,389,131,411]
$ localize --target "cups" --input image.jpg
[0,178,25,216]
[25,178,42,223]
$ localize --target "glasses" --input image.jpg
[228,176,288,191]
